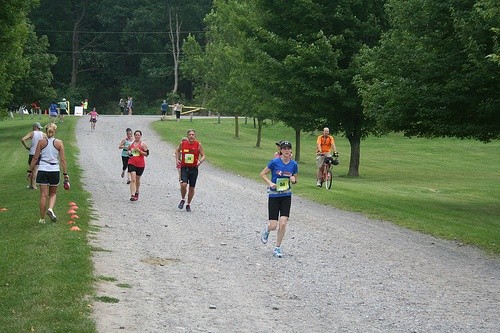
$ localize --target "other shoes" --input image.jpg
[317,181,321,187]
[127,180,131,184]
[47,209,57,223]
[121,172,125,178]
[186,205,191,212]
[130,196,134,201]
[179,200,185,209]
[39,218,45,224]
[27,185,37,189]
[134,191,139,200]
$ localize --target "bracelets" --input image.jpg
[63,173,68,175]
[27,170,33,173]
[292,181,296,184]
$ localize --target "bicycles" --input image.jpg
[316,151,340,190]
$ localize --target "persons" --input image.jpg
[50,101,59,123]
[27,123,71,225]
[175,101,181,122]
[119,128,135,184]
[87,107,99,131]
[119,96,132,115]
[175,129,205,212]
[316,127,337,186]
[82,99,88,115]
[127,130,149,201]
[21,121,46,190]
[260,141,299,259]
[58,98,67,122]
[267,141,293,193]
[32,100,40,114]
[161,99,168,121]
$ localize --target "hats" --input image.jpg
[33,122,43,129]
[281,142,292,148]
[275,140,284,145]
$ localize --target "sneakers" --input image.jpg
[274,247,282,257]
[261,228,269,244]
